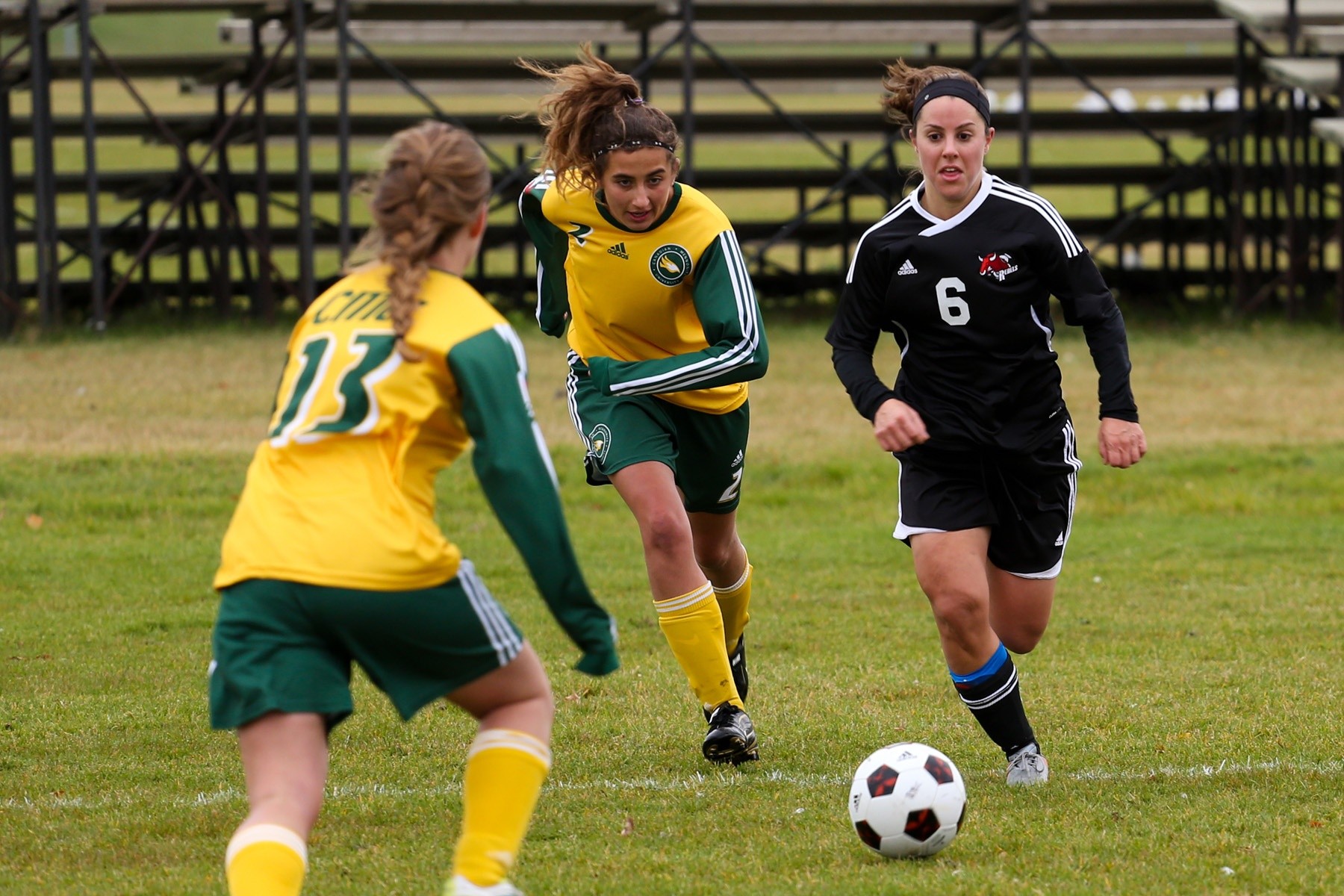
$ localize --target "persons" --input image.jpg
[819,59,1151,790]
[198,115,624,895]
[501,36,773,768]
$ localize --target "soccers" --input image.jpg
[849,742,966,860]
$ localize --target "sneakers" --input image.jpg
[1004,743,1048,790]
[729,632,749,705]
[701,702,759,766]
